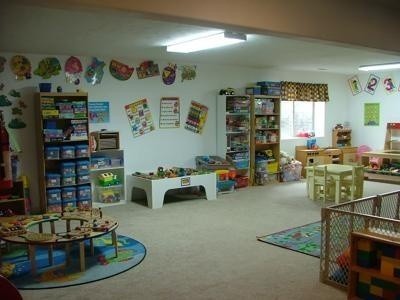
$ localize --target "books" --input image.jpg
[40,95,89,143]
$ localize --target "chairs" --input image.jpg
[302,162,365,207]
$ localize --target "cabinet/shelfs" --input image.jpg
[216,94,282,187]
[33,90,92,214]
[331,128,358,165]
[88,151,124,208]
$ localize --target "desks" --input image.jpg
[0,209,119,273]
[126,170,217,210]
[295,144,341,178]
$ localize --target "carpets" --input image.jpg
[0,234,145,290]
[256,213,355,263]
